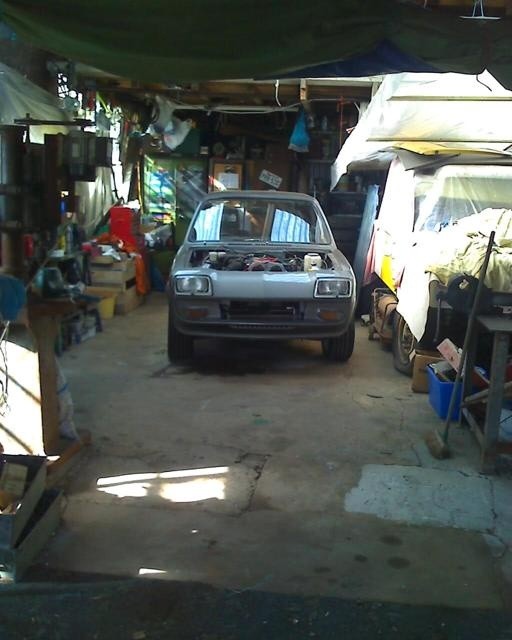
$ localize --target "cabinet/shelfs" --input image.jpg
[308,130,366,254]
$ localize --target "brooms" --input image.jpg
[424,231,496,461]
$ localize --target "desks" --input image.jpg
[459,314,512,474]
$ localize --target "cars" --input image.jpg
[165,190,358,365]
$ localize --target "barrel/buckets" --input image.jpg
[99,296,115,320]
[110,207,131,241]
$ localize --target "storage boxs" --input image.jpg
[408,348,445,393]
[424,362,490,418]
